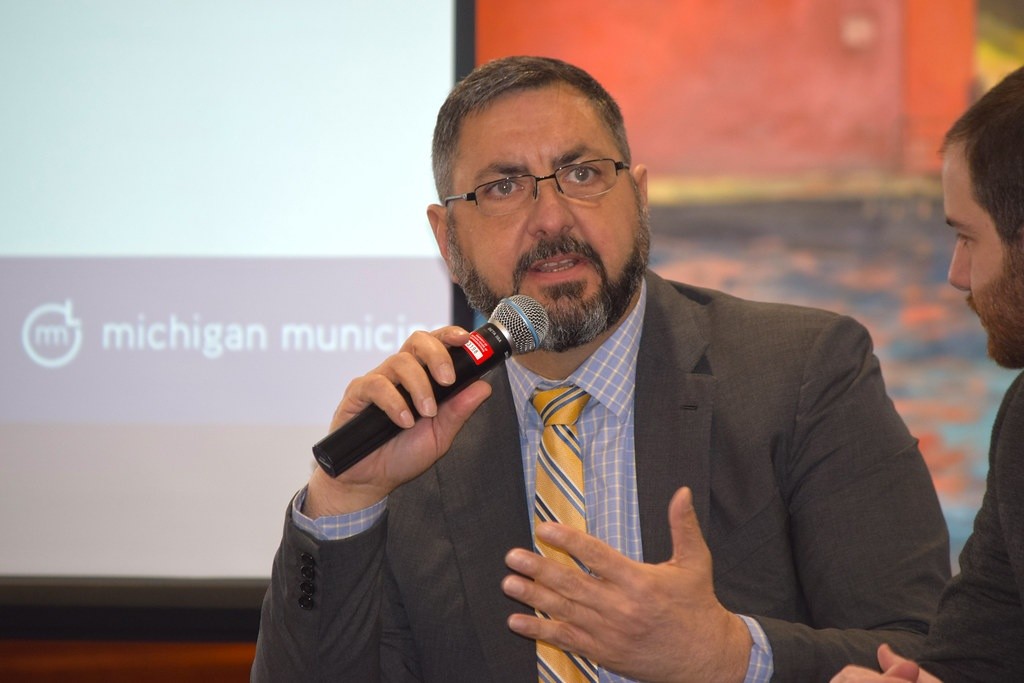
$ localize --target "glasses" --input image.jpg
[445,158,631,216]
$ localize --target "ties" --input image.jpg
[529,388,599,681]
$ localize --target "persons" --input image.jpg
[251,52,957,683]
[823,60,1023,683]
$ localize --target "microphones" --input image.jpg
[312,294,553,478]
